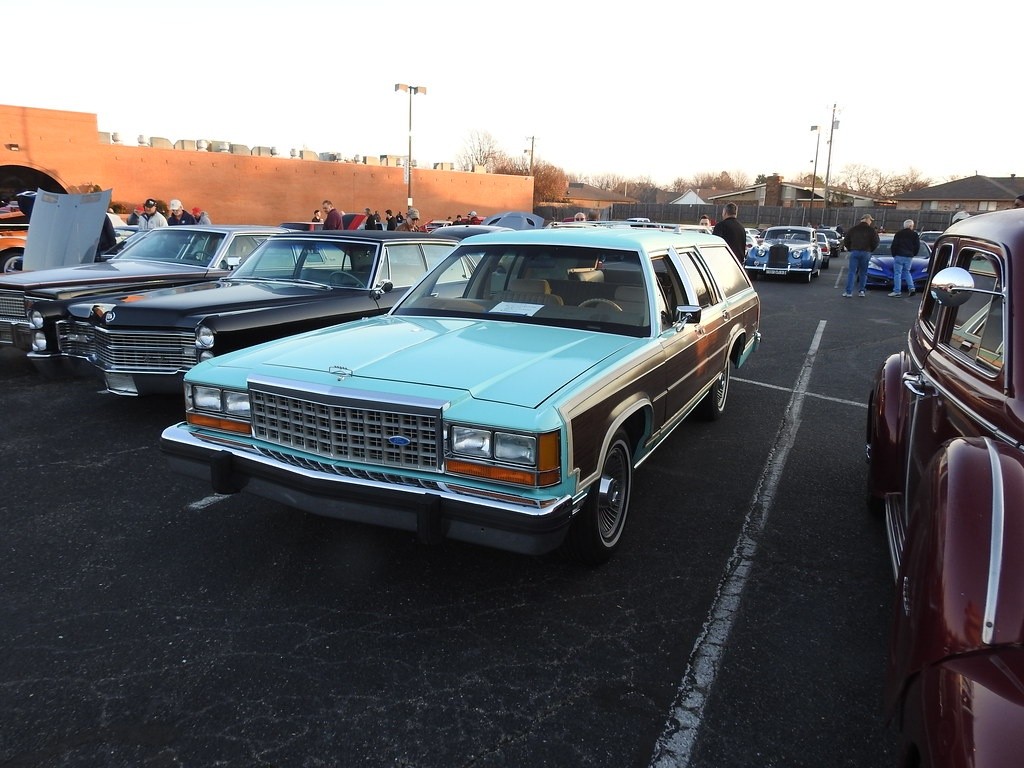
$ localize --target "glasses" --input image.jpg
[574,218,585,221]
[409,216,420,221]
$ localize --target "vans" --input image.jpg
[627,218,650,222]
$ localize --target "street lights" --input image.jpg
[395,84,426,210]
[808,126,820,222]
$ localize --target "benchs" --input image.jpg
[550,279,625,308]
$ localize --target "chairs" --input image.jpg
[493,279,563,306]
[596,285,647,316]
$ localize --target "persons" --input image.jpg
[575,209,600,222]
[313,200,420,233]
[806,223,844,237]
[887,219,920,298]
[699,202,747,267]
[95,198,210,261]
[842,214,880,297]
[1014,195,1024,209]
[952,210,974,225]
[447,211,482,225]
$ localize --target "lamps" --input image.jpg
[10,144,19,151]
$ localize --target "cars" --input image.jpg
[25,231,479,413]
[744,226,823,283]
[815,229,845,269]
[280,214,368,232]
[0,190,255,351]
[425,211,548,240]
[156,231,764,561]
[856,230,943,290]
[865,208,1024,768]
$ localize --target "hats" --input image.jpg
[952,210,973,223]
[144,199,158,207]
[407,208,420,218]
[169,199,180,209]
[134,204,144,211]
[861,214,874,221]
[192,208,200,216]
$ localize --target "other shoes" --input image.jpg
[909,289,916,298]
[888,292,901,298]
[842,292,853,297]
[858,290,865,296]
